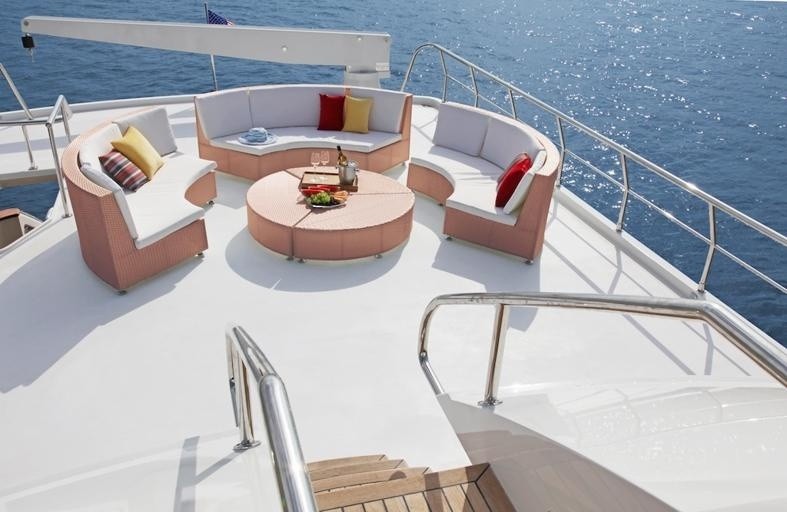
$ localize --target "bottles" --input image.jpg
[336,145,347,166]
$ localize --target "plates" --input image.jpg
[307,202,346,210]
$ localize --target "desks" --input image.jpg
[246,166,416,263]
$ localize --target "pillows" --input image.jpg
[432,102,547,215]
[194,83,409,140]
[79,124,122,172]
[98,147,149,192]
[80,164,139,240]
[112,106,178,156]
[110,124,164,181]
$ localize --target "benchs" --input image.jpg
[60,103,218,296]
[194,84,413,182]
[406,101,560,265]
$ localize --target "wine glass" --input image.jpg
[320,151,330,180]
[310,152,319,181]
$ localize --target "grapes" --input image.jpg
[311,191,330,205]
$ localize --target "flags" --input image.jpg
[208,10,235,25]
